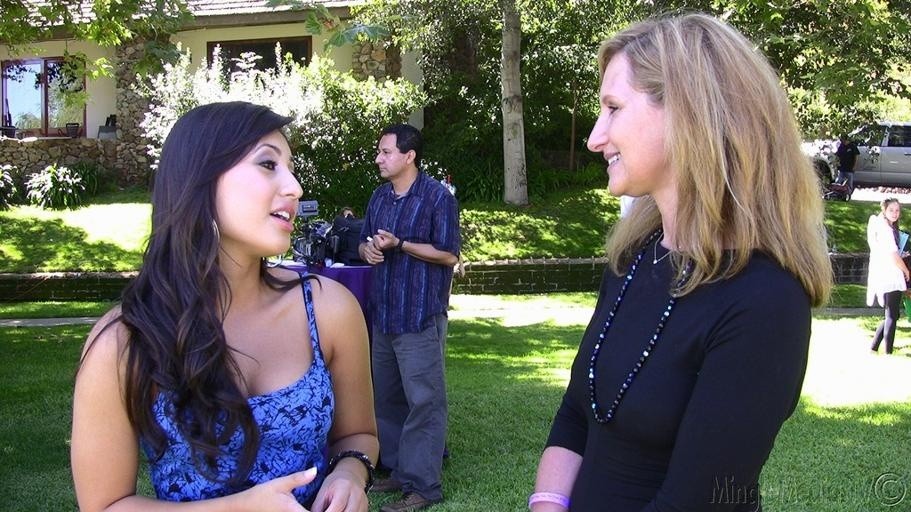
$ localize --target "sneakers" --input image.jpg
[377,488,445,512]
[369,473,408,493]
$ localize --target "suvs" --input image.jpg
[799,119,911,194]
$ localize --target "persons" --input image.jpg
[355,124,462,511]
[867,195,911,354]
[67,98,380,512]
[338,205,356,218]
[834,132,860,192]
[523,13,835,512]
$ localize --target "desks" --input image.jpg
[267,258,372,344]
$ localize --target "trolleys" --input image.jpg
[824,165,853,201]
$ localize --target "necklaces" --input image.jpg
[587,219,700,426]
[652,229,682,266]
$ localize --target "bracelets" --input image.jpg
[527,493,570,510]
[325,447,375,495]
[395,237,403,249]
